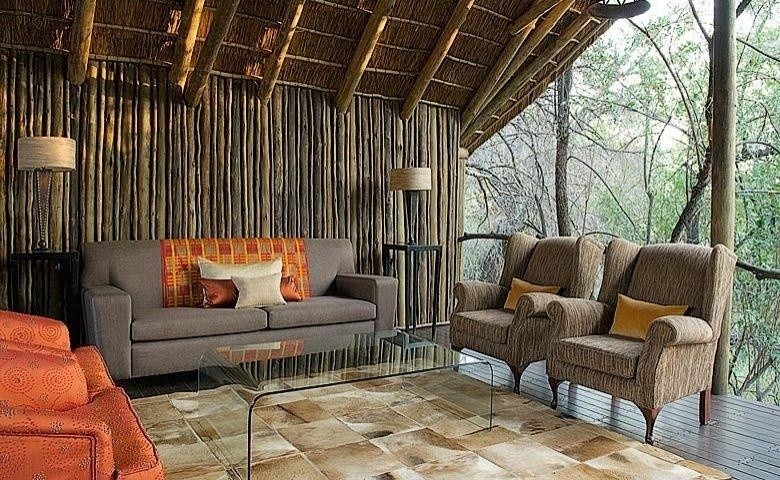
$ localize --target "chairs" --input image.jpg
[446,230,604,396]
[0,307,114,395]
[2,351,164,477]
[543,239,733,444]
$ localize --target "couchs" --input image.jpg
[78,240,393,383]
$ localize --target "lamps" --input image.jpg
[15,134,78,245]
[390,168,432,242]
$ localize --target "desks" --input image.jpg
[15,248,81,320]
[383,242,444,343]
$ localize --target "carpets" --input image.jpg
[126,358,732,480]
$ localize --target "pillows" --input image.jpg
[192,255,284,278]
[199,278,237,308]
[503,273,564,316]
[609,292,688,345]
[231,277,283,307]
